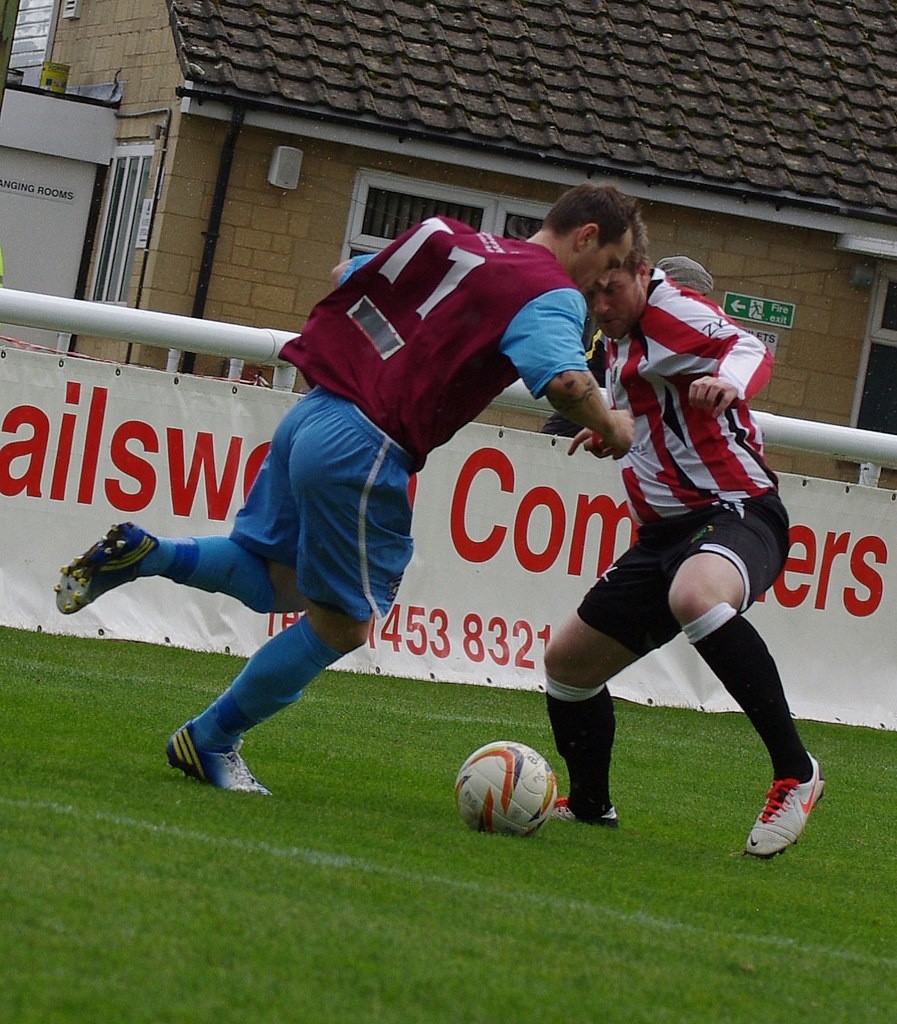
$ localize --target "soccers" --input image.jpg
[453,739,558,839]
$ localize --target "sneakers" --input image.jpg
[543,793,619,830]
[166,723,272,796]
[53,523,156,615]
[745,750,824,856]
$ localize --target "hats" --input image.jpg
[657,256,714,298]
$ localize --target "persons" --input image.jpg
[52,176,642,798]
[539,206,829,862]
[537,252,716,440]
